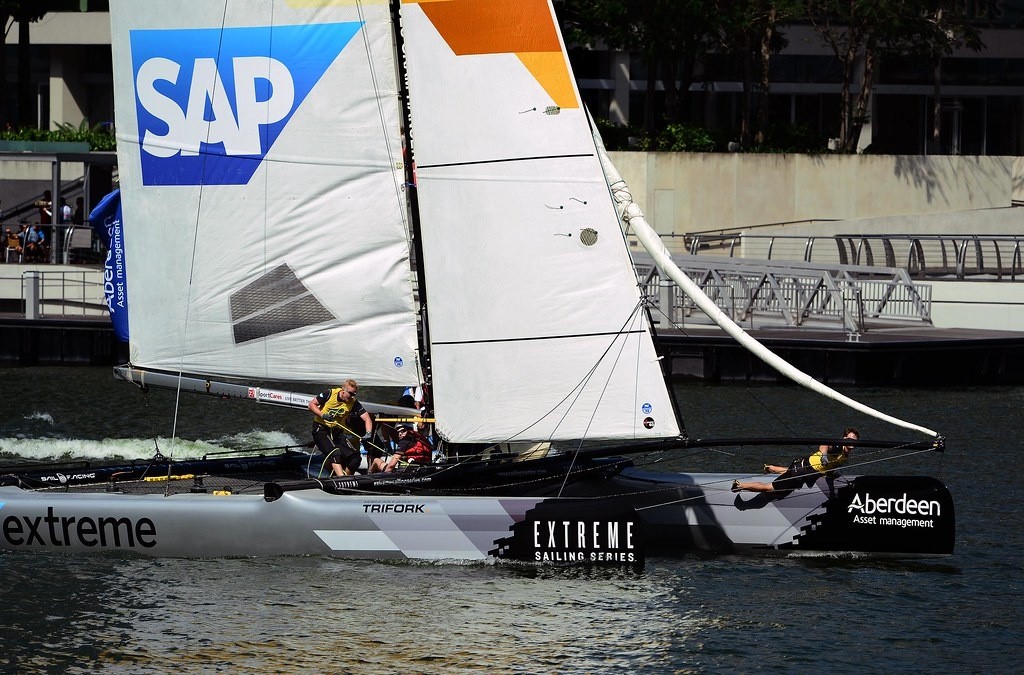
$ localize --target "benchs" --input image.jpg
[4,224,32,264]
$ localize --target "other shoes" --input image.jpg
[731,481,740,492]
[763,464,771,474]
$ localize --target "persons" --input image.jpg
[0,190,83,264]
[307,379,431,477]
[731,427,860,498]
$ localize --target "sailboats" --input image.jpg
[0,1,961,567]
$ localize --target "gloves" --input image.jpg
[322,414,334,421]
[360,433,372,444]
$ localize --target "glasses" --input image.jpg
[396,429,407,435]
[346,389,357,396]
